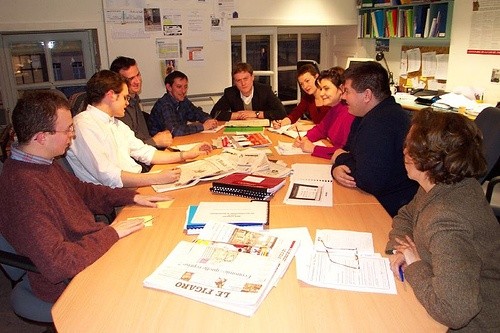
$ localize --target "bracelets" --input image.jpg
[180,151,186,162]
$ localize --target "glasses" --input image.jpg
[320,239,361,271]
[34,126,74,140]
[321,70,337,77]
[113,93,130,101]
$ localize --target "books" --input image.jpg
[358,0,448,38]
[223,119,270,133]
[181,201,270,237]
[210,173,286,202]
[219,134,272,149]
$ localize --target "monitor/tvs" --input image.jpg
[346,57,374,70]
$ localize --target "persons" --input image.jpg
[271,64,330,130]
[0,89,173,304]
[147,71,219,139]
[63,71,212,191]
[292,70,357,160]
[97,57,172,151]
[331,61,419,216]
[209,62,287,122]
[384,108,500,333]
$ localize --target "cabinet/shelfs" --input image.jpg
[357,0,454,48]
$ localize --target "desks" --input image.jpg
[401,104,476,122]
[52,205,449,333]
[125,120,380,208]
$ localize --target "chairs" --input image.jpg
[471,107,500,204]
[0,212,110,333]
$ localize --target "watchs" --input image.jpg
[255,111,259,118]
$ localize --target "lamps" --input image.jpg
[376,50,394,85]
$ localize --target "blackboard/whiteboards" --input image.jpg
[100,1,236,105]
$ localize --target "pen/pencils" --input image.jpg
[398,264,407,283]
[295,125,305,142]
